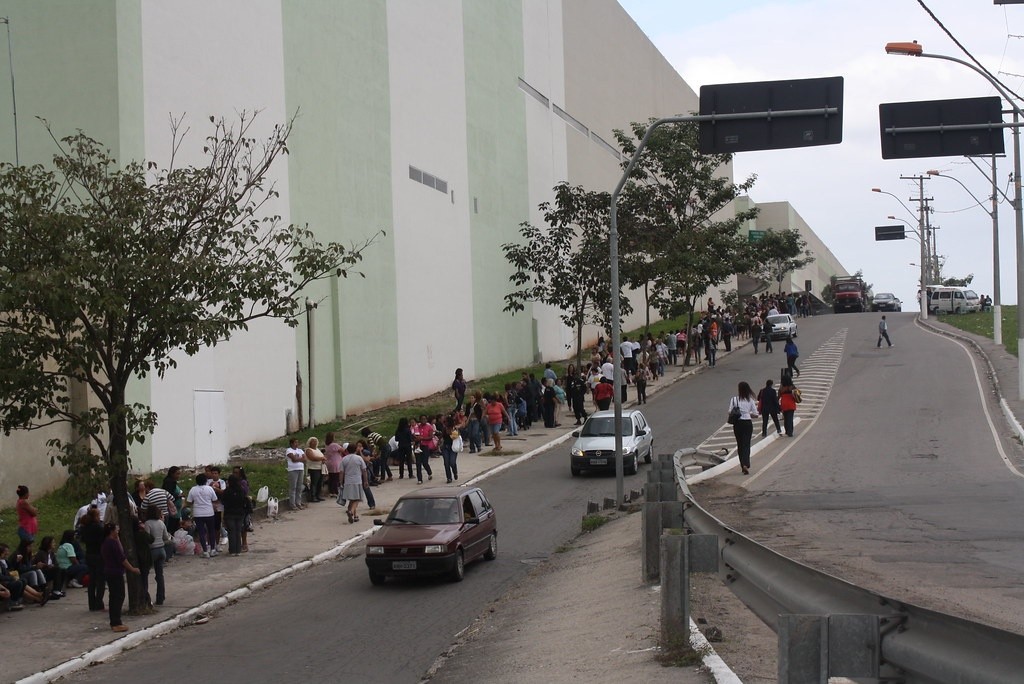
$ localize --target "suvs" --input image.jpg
[364,486,499,583]
[570,410,654,476]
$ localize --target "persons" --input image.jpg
[784,337,800,378]
[981,294,992,311]
[876,315,895,348]
[727,382,759,475]
[778,372,801,437]
[452,321,702,414]
[758,379,782,437]
[701,291,809,367]
[918,290,931,314]
[0,464,257,632]
[286,396,514,524]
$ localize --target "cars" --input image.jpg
[760,313,798,342]
[895,298,903,312]
[871,293,897,312]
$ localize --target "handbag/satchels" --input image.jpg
[452,434,464,453]
[256,486,270,503]
[321,460,329,475]
[791,384,803,404]
[756,389,764,415]
[336,487,347,506]
[267,497,279,518]
[726,396,742,425]
[465,419,470,428]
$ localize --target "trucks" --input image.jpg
[832,275,868,312]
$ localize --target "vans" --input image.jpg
[929,287,981,315]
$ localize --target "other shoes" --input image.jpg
[310,497,326,502]
[369,474,458,486]
[741,466,750,475]
[153,600,165,606]
[5,578,84,611]
[199,537,250,558]
[778,432,783,437]
[785,432,794,437]
[111,624,130,632]
[329,493,339,499]
[499,416,590,437]
[345,509,359,524]
[762,434,767,439]
[291,504,305,512]
[430,443,502,459]
[369,502,376,510]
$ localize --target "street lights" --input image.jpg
[871,187,928,319]
[887,215,927,312]
[885,41,1024,401]
